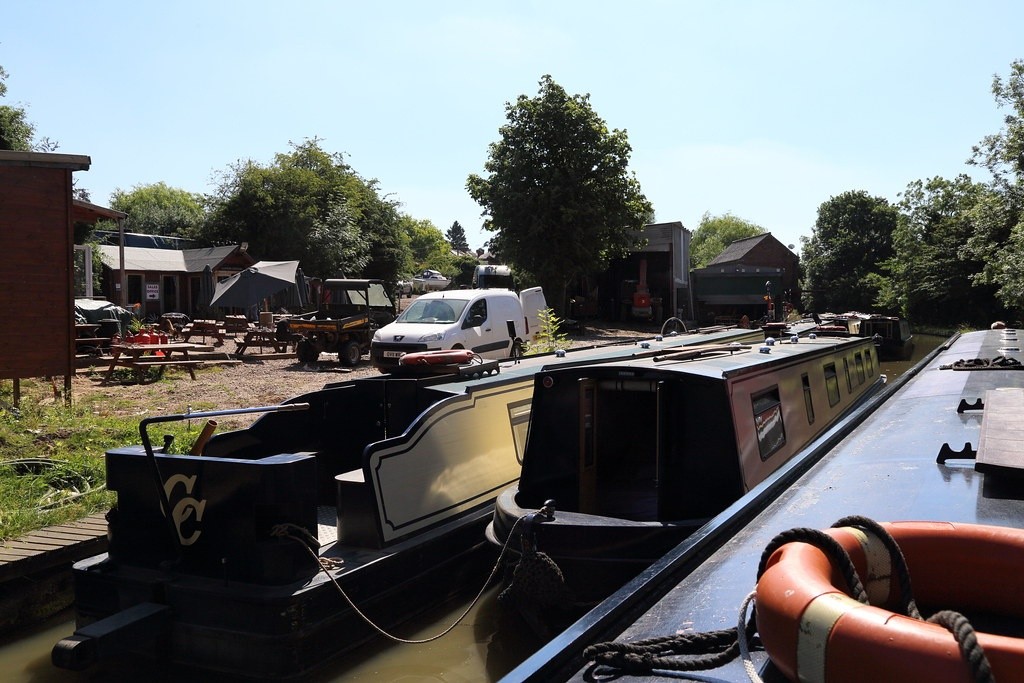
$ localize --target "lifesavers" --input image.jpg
[817,325,847,330]
[786,303,793,313]
[754,516,1023,683]
[400,348,475,366]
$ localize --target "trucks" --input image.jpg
[275,276,401,369]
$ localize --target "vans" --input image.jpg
[471,265,516,291]
[368,285,552,375]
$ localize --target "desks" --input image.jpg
[101,343,196,386]
[193,319,216,344]
[235,328,281,355]
[226,314,246,332]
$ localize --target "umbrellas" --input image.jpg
[209,261,299,309]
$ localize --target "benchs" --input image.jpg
[100,356,166,365]
[218,329,226,337]
[216,321,225,328]
[134,360,205,371]
[186,323,194,329]
[248,323,256,328]
[234,337,287,347]
[181,328,190,335]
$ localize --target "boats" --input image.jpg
[480,331,890,642]
[858,314,915,360]
[49,320,770,683]
